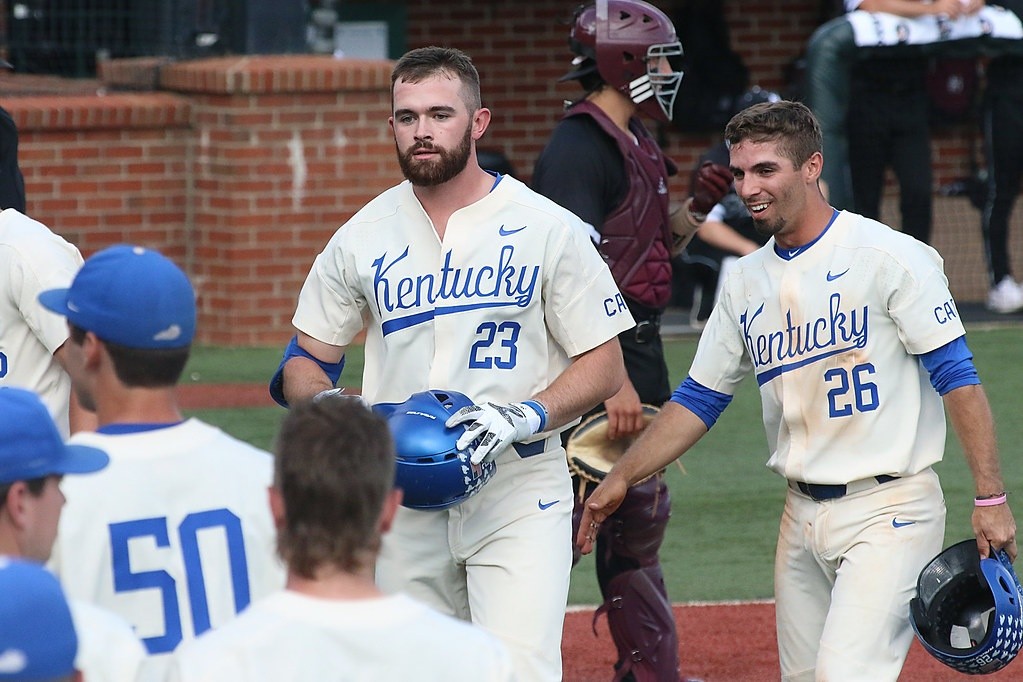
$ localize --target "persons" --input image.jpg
[0,107,98,440]
[166,391,556,682]
[0,387,111,566]
[268,47,635,682]
[0,552,84,682]
[38,245,288,682]
[847,0,1023,313]
[526,0,735,682]
[576,97,1018,682]
[685,86,828,327]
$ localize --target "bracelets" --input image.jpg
[974,492,1006,506]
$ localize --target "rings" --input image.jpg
[595,525,598,528]
[586,535,591,540]
[591,523,594,527]
[590,538,592,542]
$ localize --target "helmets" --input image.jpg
[908,538,1022,675]
[371,390,496,511]
[569,0,683,123]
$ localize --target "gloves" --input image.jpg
[445,401,550,465]
[688,160,734,215]
[311,387,372,413]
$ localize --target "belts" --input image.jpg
[617,318,661,344]
[512,439,545,459]
[788,474,902,501]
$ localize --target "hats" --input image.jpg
[0,387,109,482]
[558,46,596,82]
[734,91,782,114]
[39,245,196,349]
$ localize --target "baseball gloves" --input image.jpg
[564,403,690,521]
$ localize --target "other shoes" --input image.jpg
[987,276,1023,313]
